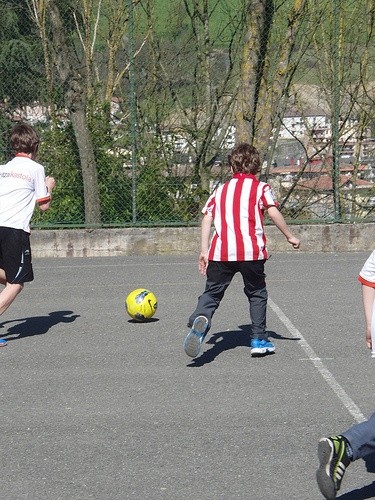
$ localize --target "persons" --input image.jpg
[183,144,300,358]
[0,125,56,347]
[316,248,375,500]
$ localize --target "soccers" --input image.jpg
[124,286,160,323]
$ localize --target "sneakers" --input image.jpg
[250,337,275,357]
[314,432,353,500]
[184,315,209,358]
